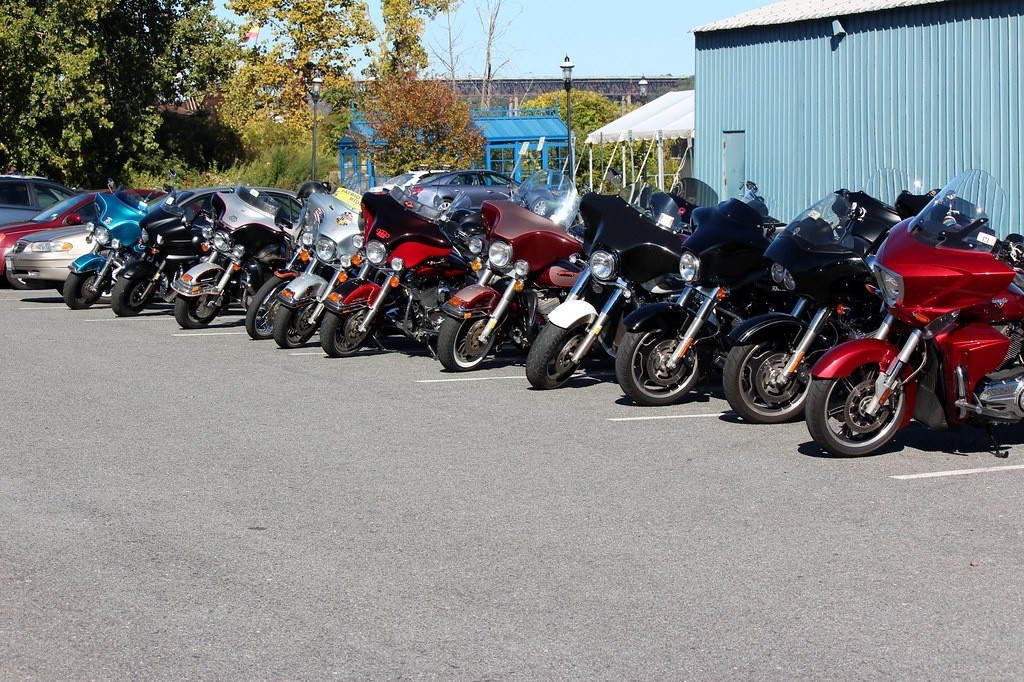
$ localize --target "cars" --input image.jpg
[0,174,80,227]
[0,188,204,285]
[364,169,445,198]
[3,184,308,302]
[397,169,521,213]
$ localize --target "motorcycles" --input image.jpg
[63,163,1023,463]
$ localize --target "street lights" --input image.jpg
[557,54,576,192]
[637,74,650,183]
